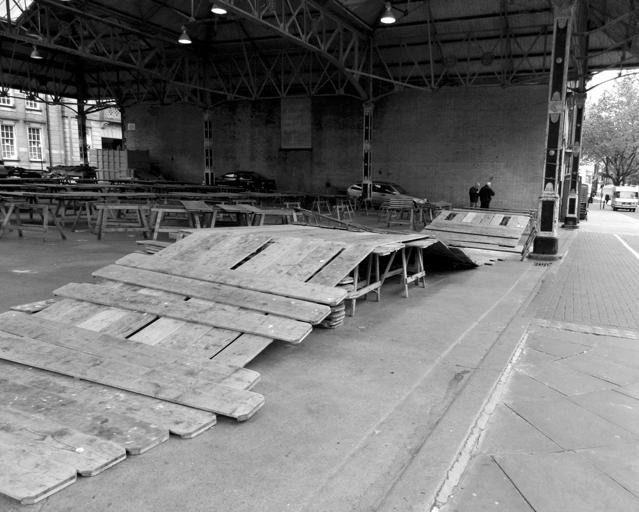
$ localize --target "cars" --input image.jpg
[215,171,277,193]
[0,164,98,184]
[347,180,429,211]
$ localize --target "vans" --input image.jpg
[610,185,638,212]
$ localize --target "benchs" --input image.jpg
[1,166,454,312]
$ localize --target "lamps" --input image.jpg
[210,0,231,16]
[380,0,398,25]
[27,43,44,60]
[177,21,194,45]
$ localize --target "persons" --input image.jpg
[0,160,8,174]
[479,182,495,208]
[469,181,480,207]
